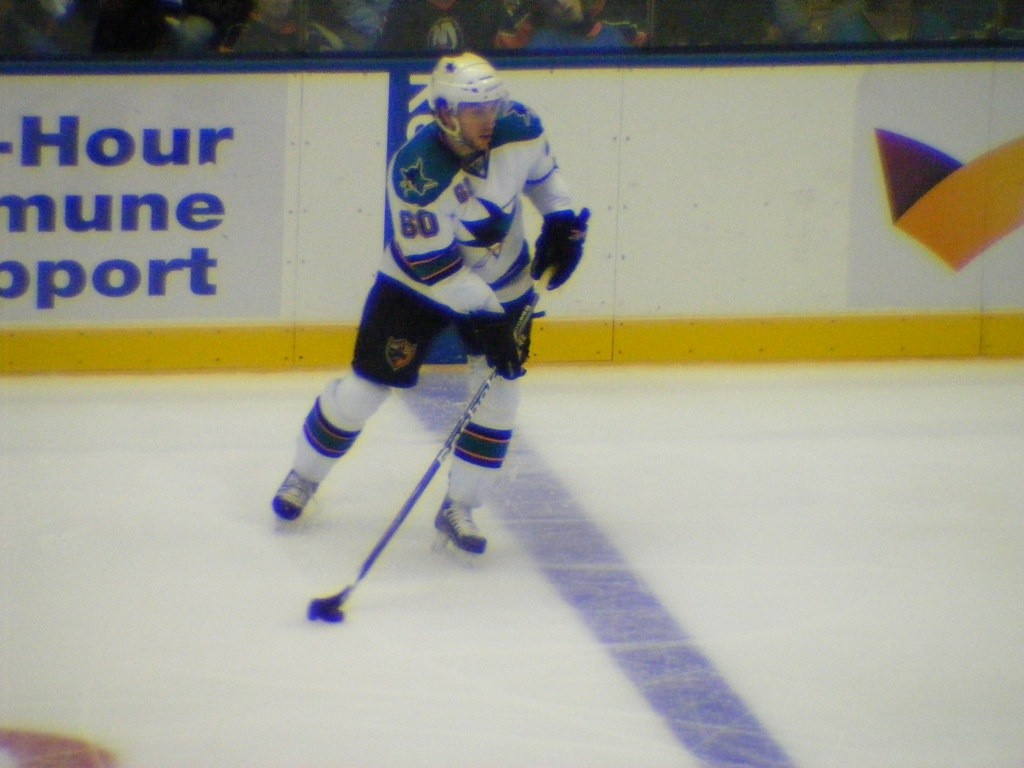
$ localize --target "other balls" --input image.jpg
[308,608,344,622]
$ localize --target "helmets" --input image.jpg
[428,51,505,139]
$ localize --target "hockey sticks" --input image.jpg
[306,258,563,610]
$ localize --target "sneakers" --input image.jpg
[271,469,320,531]
[433,471,487,569]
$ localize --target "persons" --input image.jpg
[0,0,1024,61]
[273,52,590,554]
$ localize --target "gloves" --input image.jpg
[530,208,590,290]
[477,323,526,379]
